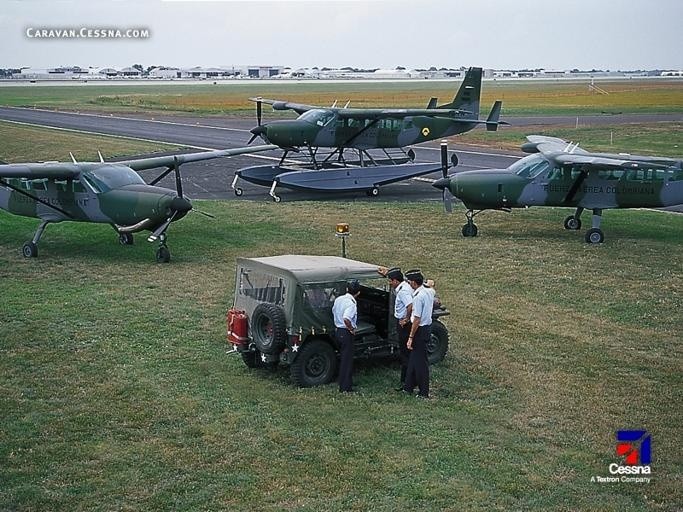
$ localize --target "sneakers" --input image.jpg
[396,389,411,395]
[414,393,428,398]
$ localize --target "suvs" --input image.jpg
[224,221,454,388]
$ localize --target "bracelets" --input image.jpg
[350,328,354,331]
[408,335,412,338]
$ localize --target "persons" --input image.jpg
[404,269,436,399]
[377,265,415,393]
[331,281,362,394]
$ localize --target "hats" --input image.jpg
[350,279,360,290]
[387,268,402,278]
[404,270,422,278]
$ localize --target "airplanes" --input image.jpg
[229,66,511,173]
[431,134,682,246]
[0,141,281,262]
